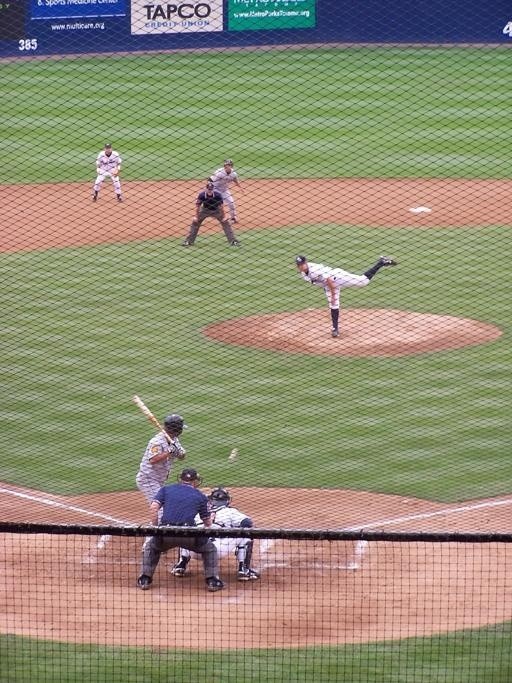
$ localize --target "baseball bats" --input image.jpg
[133,395,177,449]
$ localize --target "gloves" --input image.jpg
[168,445,186,458]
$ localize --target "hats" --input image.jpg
[207,183,214,190]
[295,255,306,265]
[105,142,111,148]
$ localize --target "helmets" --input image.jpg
[164,414,183,433]
[224,159,233,165]
[211,488,229,500]
[180,469,198,481]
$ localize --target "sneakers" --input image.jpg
[136,578,151,590]
[330,326,339,338]
[116,194,122,202]
[231,241,240,246]
[182,241,193,246]
[170,565,185,577]
[207,576,227,592]
[90,195,98,202]
[237,569,259,581]
[380,255,397,266]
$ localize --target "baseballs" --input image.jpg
[227,447,238,463]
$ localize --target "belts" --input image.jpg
[159,523,195,527]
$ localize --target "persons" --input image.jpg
[135,413,189,552]
[169,485,261,582]
[135,467,227,593]
[210,158,248,222]
[294,252,398,337]
[181,181,243,247]
[91,142,125,203]
